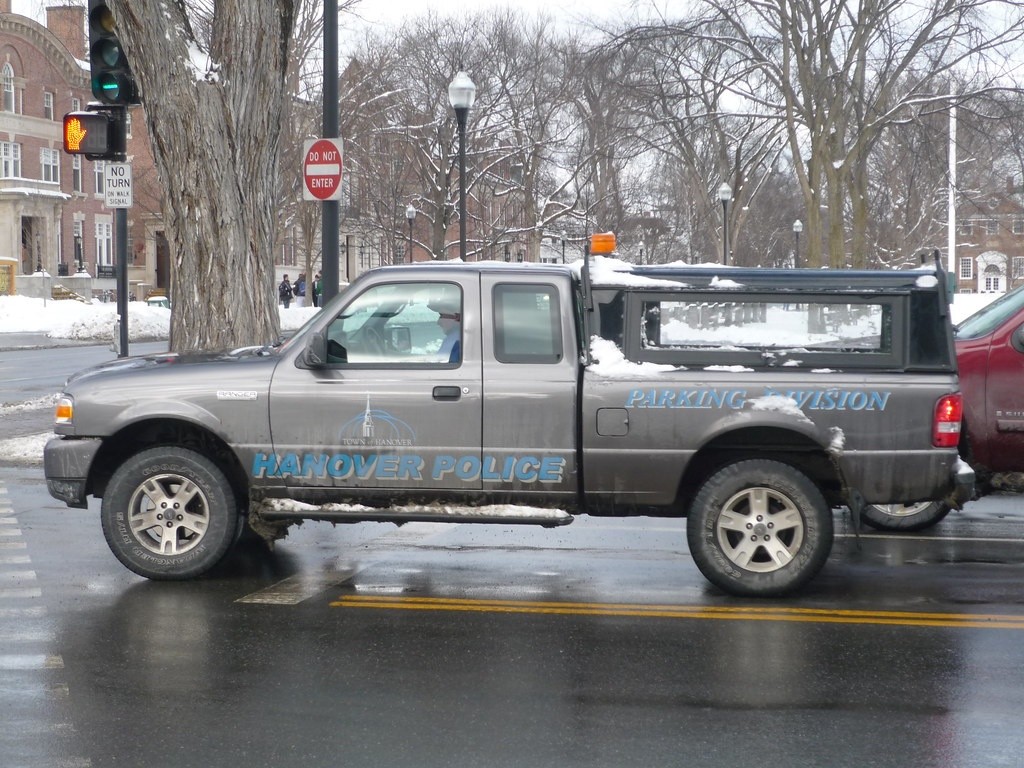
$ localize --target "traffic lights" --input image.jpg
[62,112,114,158]
[88,1,138,106]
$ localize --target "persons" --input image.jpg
[311,274,320,307]
[426,287,461,365]
[294,272,305,296]
[317,270,323,307]
[279,274,293,309]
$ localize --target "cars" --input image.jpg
[847,285,1024,530]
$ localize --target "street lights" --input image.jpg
[76,234,86,273]
[793,218,804,268]
[718,179,731,265]
[448,63,476,261]
[406,204,417,263]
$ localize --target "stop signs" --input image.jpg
[304,137,344,200]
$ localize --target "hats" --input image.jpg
[426,286,460,313]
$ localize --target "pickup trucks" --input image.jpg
[42,233,980,602]
